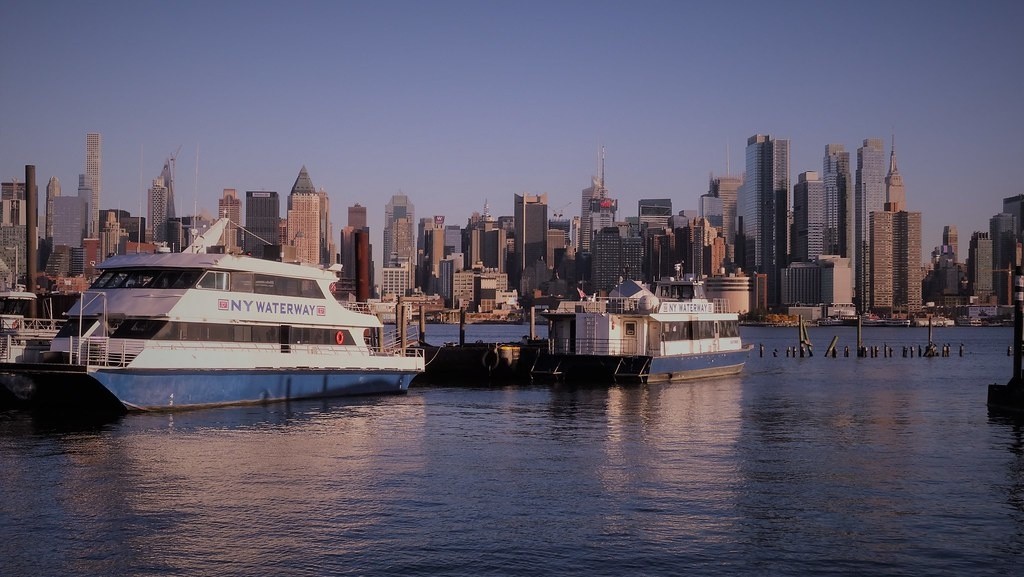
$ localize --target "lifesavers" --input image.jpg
[335,330,344,344]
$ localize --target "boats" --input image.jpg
[816,318,843,326]
[862,317,911,327]
[528,287,753,392]
[1,216,425,416]
